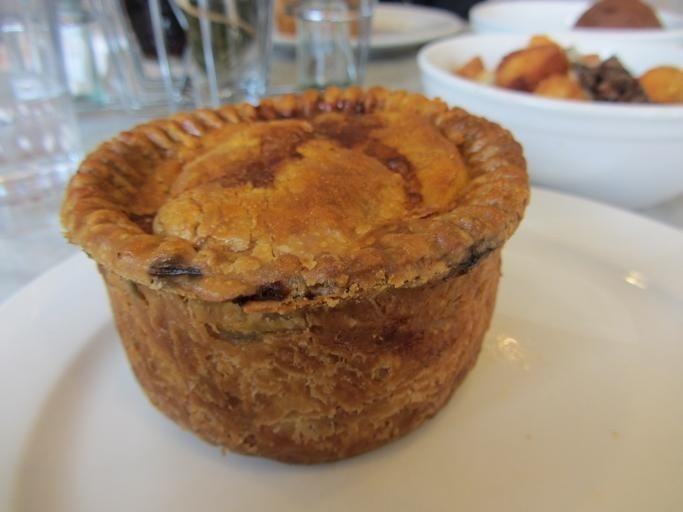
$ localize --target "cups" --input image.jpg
[0,0,369,209]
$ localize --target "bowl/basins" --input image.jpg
[416,31,681,208]
[468,1,680,35]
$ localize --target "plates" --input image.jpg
[261,3,463,51]
[1,182,683,512]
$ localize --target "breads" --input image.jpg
[273,1,361,38]
[60,84,530,462]
[457,34,683,105]
[570,0,664,30]
